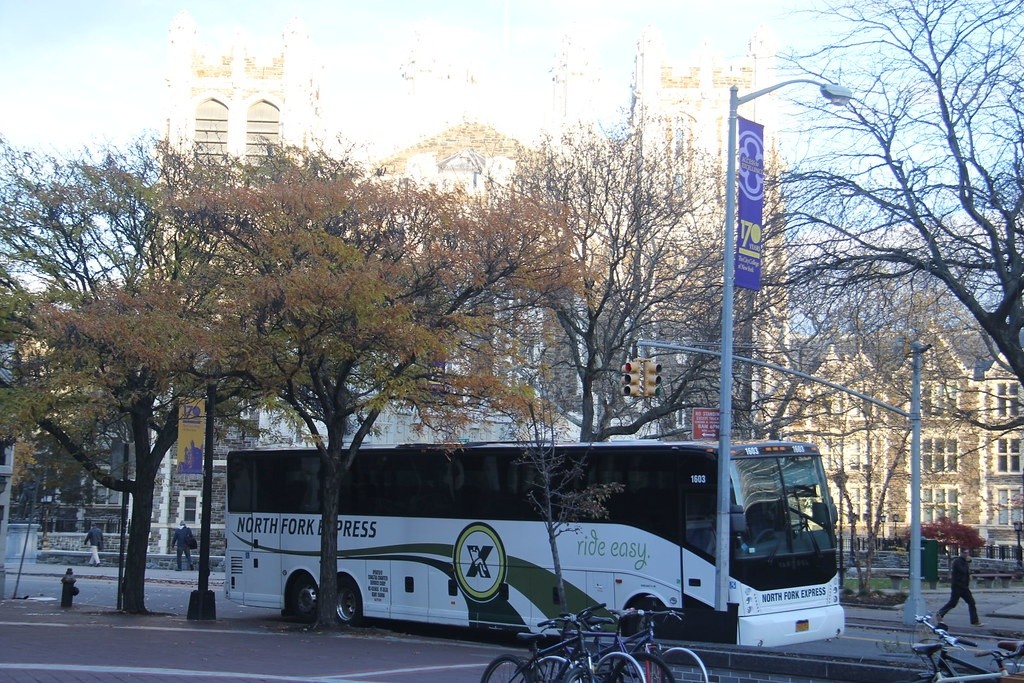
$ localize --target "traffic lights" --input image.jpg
[624,361,640,398]
[620,364,627,398]
[643,360,663,399]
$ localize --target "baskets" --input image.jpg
[917,638,945,668]
[620,613,646,637]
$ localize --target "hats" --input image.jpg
[92,524,96,526]
[180,520,185,525]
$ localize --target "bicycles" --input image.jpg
[905,612,1024,683]
[910,640,1024,683]
[478,599,709,683]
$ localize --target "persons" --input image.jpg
[172,519,196,571]
[936,546,985,628]
[84,523,104,567]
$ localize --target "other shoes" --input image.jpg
[936,611,944,623]
[190,563,194,570]
[970,622,984,627]
[175,568,181,571]
[94,563,99,567]
[89,564,92,567]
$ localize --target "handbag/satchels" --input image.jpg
[98,541,103,550]
[190,539,197,549]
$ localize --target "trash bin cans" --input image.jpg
[905,538,939,590]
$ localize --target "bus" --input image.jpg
[224,438,847,649]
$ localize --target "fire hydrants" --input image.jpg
[59,567,79,608]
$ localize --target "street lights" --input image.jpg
[37,491,54,550]
[711,77,855,643]
[1012,520,1024,573]
[892,514,899,538]
[880,514,886,541]
[847,512,856,557]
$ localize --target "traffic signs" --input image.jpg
[691,407,719,442]
